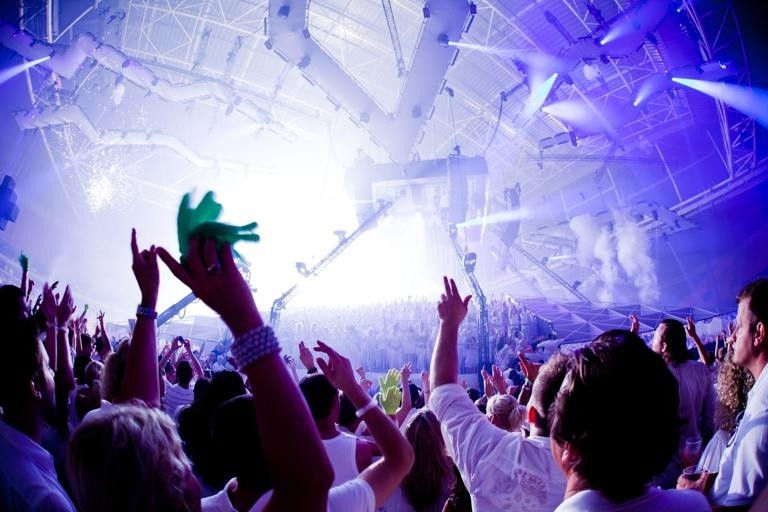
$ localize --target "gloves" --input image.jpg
[176,191,259,270]
[379,368,401,398]
[380,386,402,415]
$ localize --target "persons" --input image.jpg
[1,227,767,511]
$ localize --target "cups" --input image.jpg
[686,436,702,454]
[683,465,709,481]
